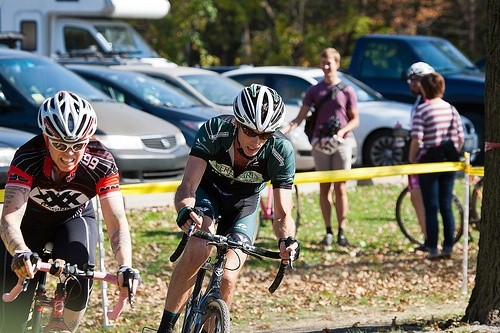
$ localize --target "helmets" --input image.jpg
[406,62,435,79]
[38,91,98,143]
[233,83,286,132]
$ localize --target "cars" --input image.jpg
[0,47,190,180]
[0,126,39,181]
[64,64,233,150]
[220,65,480,167]
[113,65,356,172]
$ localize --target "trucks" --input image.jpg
[0,0,173,64]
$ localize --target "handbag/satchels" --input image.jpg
[304,110,317,144]
[423,140,460,180]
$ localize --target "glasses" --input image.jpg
[239,121,275,140]
[407,76,422,84]
[47,137,89,153]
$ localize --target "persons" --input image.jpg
[0,91,142,333]
[406,62,436,253]
[156,84,300,333]
[284,48,359,246]
[410,72,465,259]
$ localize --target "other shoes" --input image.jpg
[338,233,349,247]
[319,234,335,246]
[427,247,452,260]
[414,242,438,256]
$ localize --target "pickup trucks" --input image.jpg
[340,34,485,131]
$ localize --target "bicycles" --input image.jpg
[395,147,482,251]
[0,253,135,333]
[169,199,298,333]
